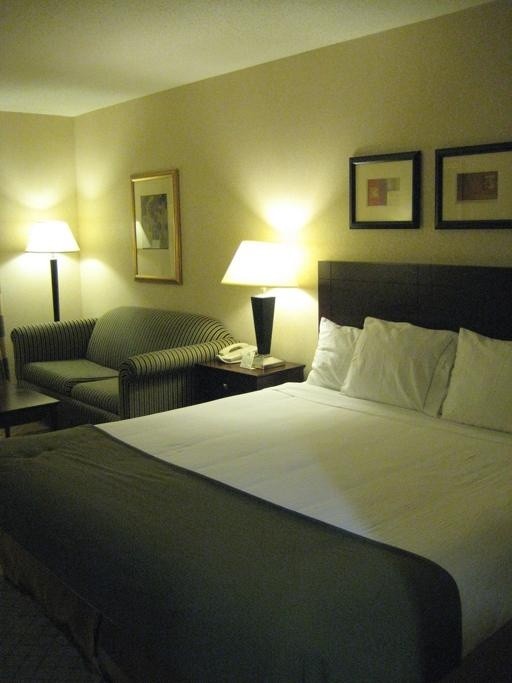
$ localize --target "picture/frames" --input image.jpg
[347,147,423,231]
[128,166,184,285]
[432,139,511,232]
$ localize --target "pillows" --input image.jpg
[305,316,364,391]
[440,324,512,434]
[337,314,459,418]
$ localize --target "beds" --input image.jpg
[0,258,512,681]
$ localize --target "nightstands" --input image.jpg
[193,357,307,405]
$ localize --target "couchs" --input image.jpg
[9,301,241,429]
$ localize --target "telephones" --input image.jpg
[218,343,258,362]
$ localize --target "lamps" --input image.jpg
[21,219,82,322]
[220,235,305,358]
